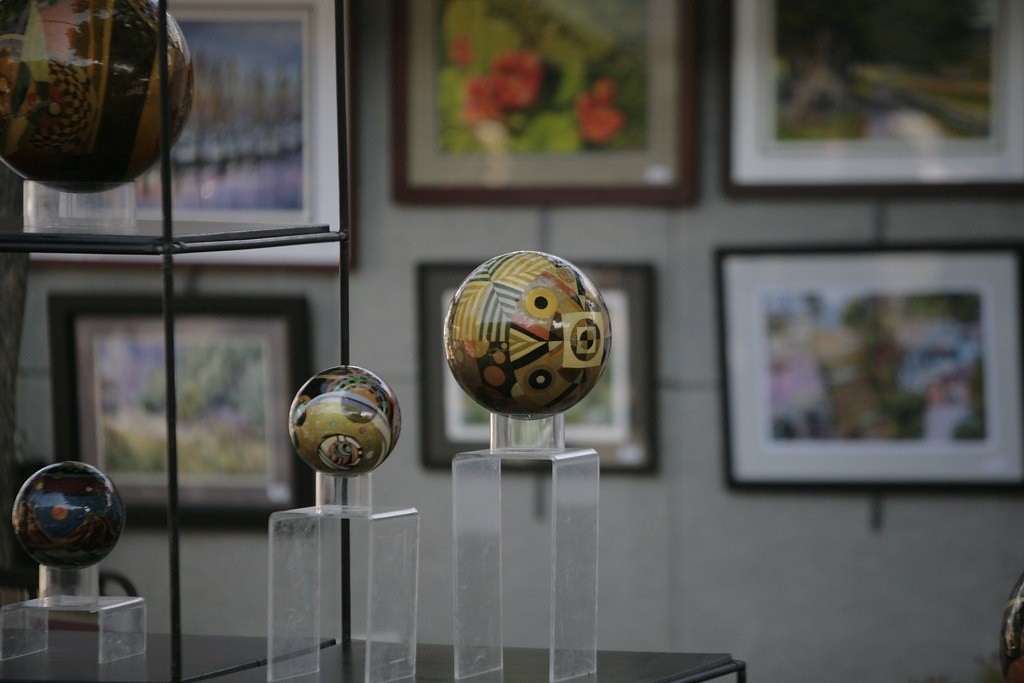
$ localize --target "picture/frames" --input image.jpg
[417,258,661,477]
[726,2,1023,197]
[46,289,316,534]
[713,241,1024,497]
[22,1,352,269]
[390,0,705,209]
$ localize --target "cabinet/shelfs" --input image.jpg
[0,0,747,683]
